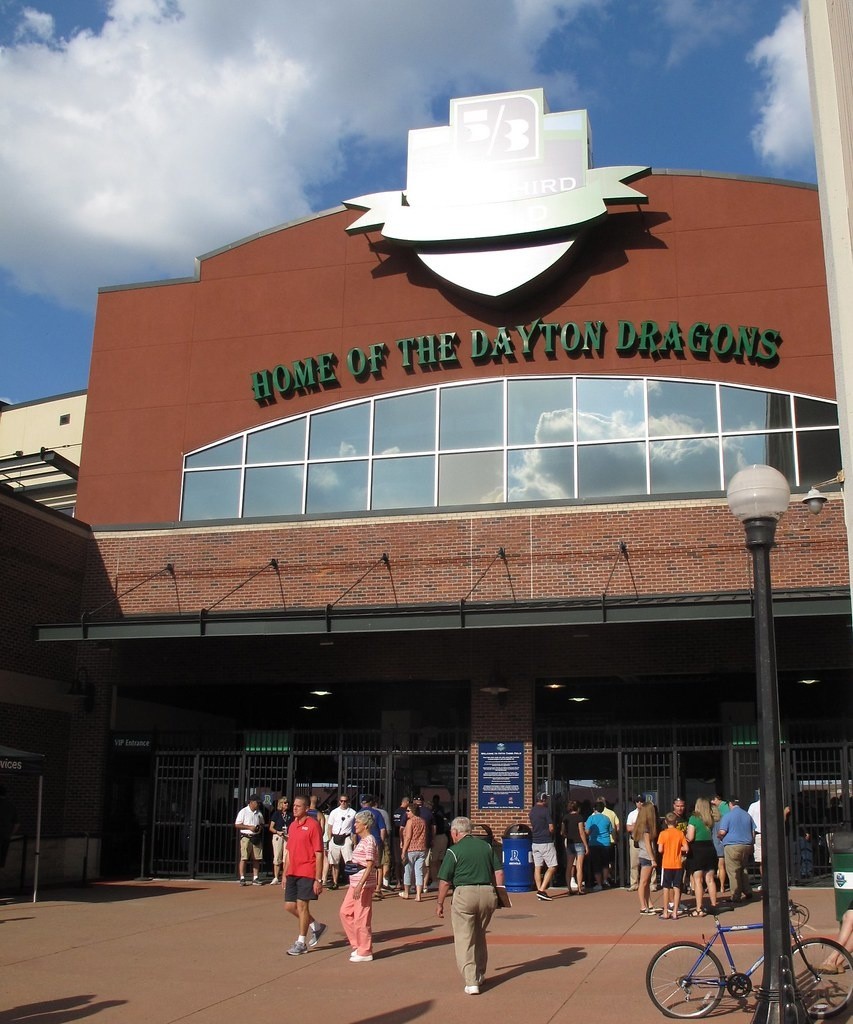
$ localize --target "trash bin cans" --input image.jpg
[828,833,853,921]
[471,824,495,847]
[500,824,534,893]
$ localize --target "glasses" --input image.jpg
[636,800,643,803]
[407,810,412,812]
[340,800,347,802]
[284,802,288,804]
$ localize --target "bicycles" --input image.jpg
[645,898,853,1021]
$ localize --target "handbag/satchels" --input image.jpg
[332,834,353,846]
[345,860,365,875]
[243,835,261,845]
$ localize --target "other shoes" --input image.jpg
[639,908,656,916]
[649,907,664,912]
[349,949,373,962]
[815,964,838,975]
[373,890,385,900]
[270,878,278,885]
[465,985,479,996]
[252,879,263,886]
[240,879,247,886]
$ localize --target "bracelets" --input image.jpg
[314,878,323,884]
[359,882,363,886]
[437,900,443,905]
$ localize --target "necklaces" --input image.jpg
[339,807,349,821]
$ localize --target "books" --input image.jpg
[495,885,513,908]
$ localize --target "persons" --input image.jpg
[784,789,853,884]
[560,796,620,895]
[372,795,436,902]
[627,792,757,920]
[235,793,266,886]
[528,791,558,901]
[432,793,448,865]
[268,794,329,956]
[325,793,387,962]
[747,787,790,895]
[437,817,504,995]
[813,899,853,974]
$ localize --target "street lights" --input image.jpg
[725,465,814,1024]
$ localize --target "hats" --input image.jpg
[249,794,262,803]
[724,795,739,805]
[634,795,643,802]
[413,795,424,801]
[536,792,550,801]
[360,795,372,804]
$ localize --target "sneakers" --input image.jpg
[537,891,553,901]
[309,923,327,946]
[286,944,308,955]
